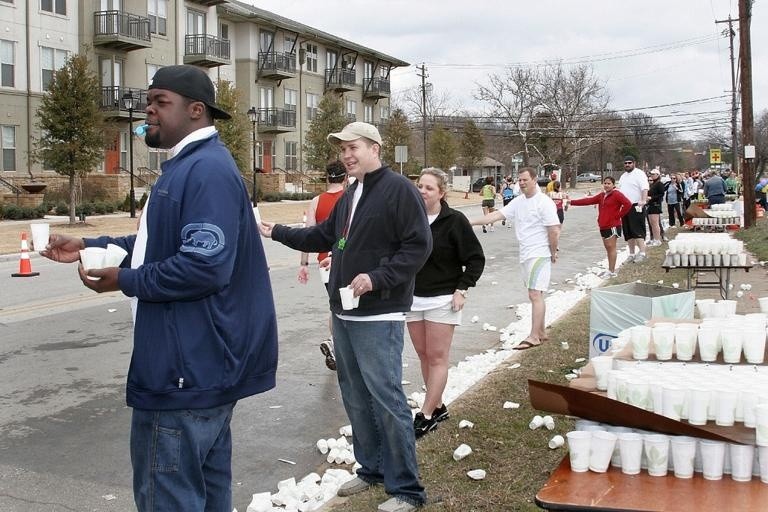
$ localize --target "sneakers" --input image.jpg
[632,255,646,262]
[645,240,662,247]
[622,255,636,265]
[319,339,337,371]
[482,225,487,233]
[411,404,448,439]
[598,270,616,279]
[378,497,417,512]
[338,476,370,496]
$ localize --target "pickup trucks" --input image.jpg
[470,177,504,193]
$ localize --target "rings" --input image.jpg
[359,286,364,291]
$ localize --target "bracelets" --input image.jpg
[300,261,310,266]
[552,253,557,256]
[328,255,334,259]
[456,288,469,300]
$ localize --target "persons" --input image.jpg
[617,155,649,264]
[27,63,279,512]
[465,166,562,351]
[404,166,485,439]
[476,170,573,237]
[566,177,632,277]
[644,167,768,248]
[256,120,434,512]
[297,159,348,370]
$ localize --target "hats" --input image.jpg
[649,169,660,175]
[623,156,634,161]
[149,67,232,121]
[326,122,383,147]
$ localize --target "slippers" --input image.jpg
[513,339,540,351]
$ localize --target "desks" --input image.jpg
[661,251,753,299]
[527,335,768,512]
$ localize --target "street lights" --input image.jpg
[121,89,140,219]
[245,106,262,224]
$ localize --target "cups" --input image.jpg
[664,231,749,267]
[635,205,643,212]
[630,295,768,484]
[29,223,50,252]
[78,243,128,280]
[658,279,752,297]
[692,218,740,224]
[710,203,737,212]
[252,206,261,225]
[232,243,630,512]
[690,199,709,203]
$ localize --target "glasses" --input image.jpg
[671,178,676,179]
[648,180,653,182]
[624,162,632,164]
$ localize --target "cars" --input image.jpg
[537,177,552,187]
[568,173,601,182]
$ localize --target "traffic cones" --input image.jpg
[11,233,42,278]
[301,210,309,229]
[463,191,470,200]
